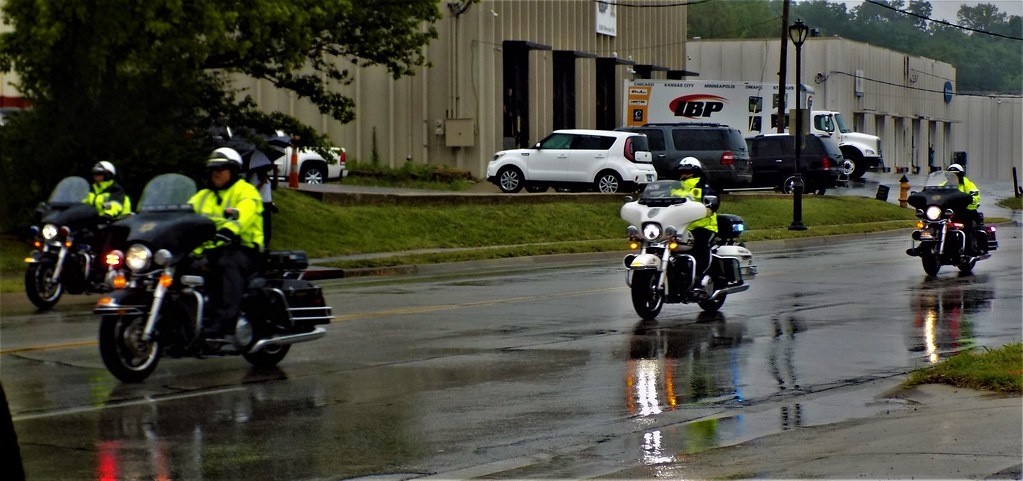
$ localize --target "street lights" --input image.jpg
[788,18,807,231]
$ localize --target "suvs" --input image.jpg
[615,122,754,194]
[485,129,658,195]
[745,132,849,196]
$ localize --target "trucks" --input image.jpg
[620,79,885,183]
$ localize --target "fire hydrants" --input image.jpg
[898,175,912,208]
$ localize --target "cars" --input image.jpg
[268,128,350,187]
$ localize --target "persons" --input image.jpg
[184,147,264,340]
[503,87,514,137]
[248,169,277,243]
[939,163,983,255]
[659,156,718,286]
[83,160,132,286]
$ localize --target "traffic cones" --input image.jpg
[288,146,299,191]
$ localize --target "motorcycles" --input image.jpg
[906,170,998,276]
[22,176,109,310]
[619,181,759,320]
[93,173,336,384]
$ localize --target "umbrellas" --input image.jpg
[238,137,291,172]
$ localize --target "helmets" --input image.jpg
[679,157,701,170]
[948,165,964,176]
[206,147,243,169]
[92,160,115,176]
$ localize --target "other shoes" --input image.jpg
[692,278,705,290]
[968,245,978,256]
[205,327,227,339]
